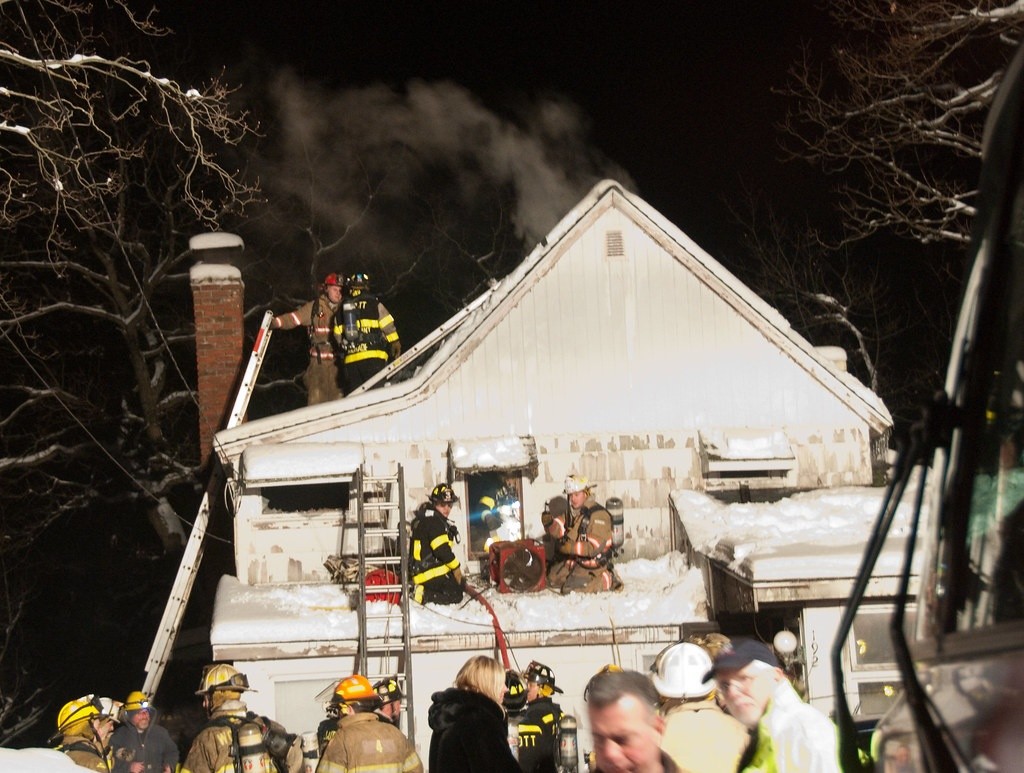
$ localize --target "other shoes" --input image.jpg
[610,567,625,592]
[414,586,426,604]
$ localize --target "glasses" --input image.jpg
[90,696,105,711]
[440,502,453,509]
[215,674,249,687]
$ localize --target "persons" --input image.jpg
[476,470,523,551]
[542,475,624,595]
[108,690,179,773]
[407,484,466,605]
[271,272,346,404]
[429,655,525,773]
[179,664,304,773]
[333,273,402,398]
[47,693,121,773]
[702,640,843,773]
[524,660,565,773]
[650,633,750,773]
[583,666,693,773]
[314,674,426,773]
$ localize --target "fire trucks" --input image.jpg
[831,47,1024,773]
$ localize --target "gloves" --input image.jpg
[542,511,553,527]
[557,536,569,552]
[259,317,276,330]
[391,341,401,359]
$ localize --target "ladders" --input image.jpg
[355,463,415,750]
[143,310,274,704]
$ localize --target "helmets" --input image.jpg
[503,671,526,710]
[124,691,152,711]
[100,697,125,724]
[57,699,101,732]
[523,662,564,694]
[331,674,378,702]
[565,475,590,494]
[347,273,369,290]
[428,483,458,503]
[195,664,259,695]
[372,679,407,711]
[652,642,715,699]
[326,272,344,286]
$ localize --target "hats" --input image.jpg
[700,638,783,685]
